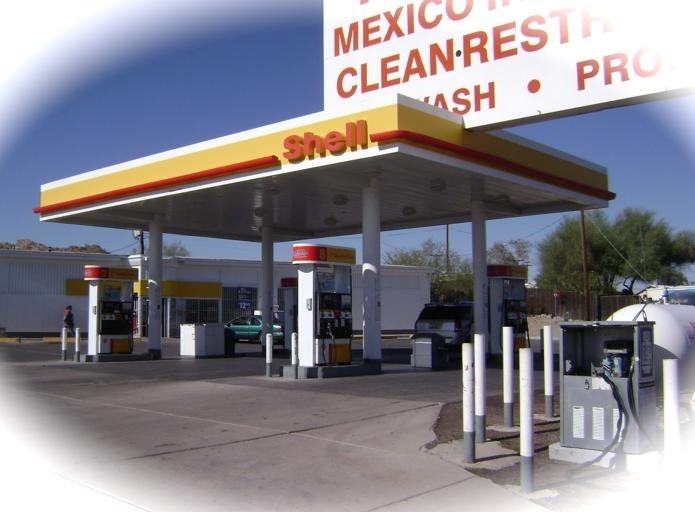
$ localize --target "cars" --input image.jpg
[226,313,287,345]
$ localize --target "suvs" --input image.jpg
[414,300,474,352]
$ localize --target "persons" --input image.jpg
[61,305,75,338]
